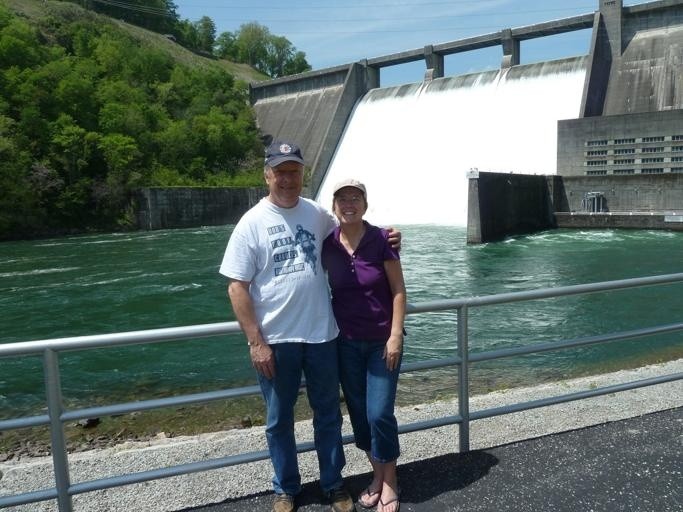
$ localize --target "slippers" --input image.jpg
[377,486,399,512]
[360,483,380,508]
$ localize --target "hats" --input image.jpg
[264,143,303,167]
[333,179,366,201]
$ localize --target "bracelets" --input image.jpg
[246,335,262,348]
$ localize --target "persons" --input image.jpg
[321,178,407,512]
[214,140,398,511]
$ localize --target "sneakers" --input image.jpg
[271,492,293,512]
[326,485,356,512]
